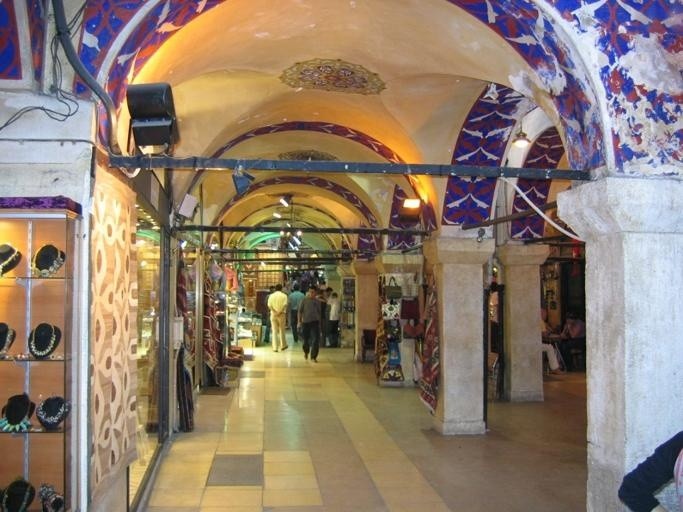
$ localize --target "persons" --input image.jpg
[561,310,585,363]
[263,269,341,362]
[618,428,683,512]
[541,308,561,375]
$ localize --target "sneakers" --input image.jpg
[272,340,339,362]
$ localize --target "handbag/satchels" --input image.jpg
[374,276,425,383]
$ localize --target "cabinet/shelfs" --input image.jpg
[0,195,79,512]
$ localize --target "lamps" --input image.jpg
[126,83,181,156]
[398,198,422,222]
[280,194,292,208]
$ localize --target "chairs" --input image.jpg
[540,329,586,377]
